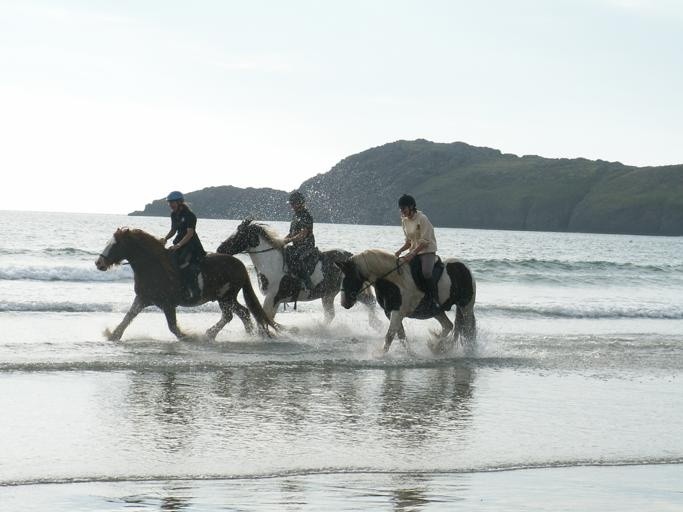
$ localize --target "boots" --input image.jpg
[424,278,432,311]
[302,264,310,280]
[180,262,196,299]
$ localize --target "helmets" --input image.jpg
[290,192,305,204]
[166,191,184,201]
[399,194,416,208]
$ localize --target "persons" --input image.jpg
[158,191,206,301]
[394,192,438,302]
[271,192,319,296]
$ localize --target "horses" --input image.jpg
[94,224,288,343]
[331,247,477,356]
[215,217,386,339]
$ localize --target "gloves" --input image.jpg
[168,243,181,251]
[159,238,167,245]
[279,238,290,247]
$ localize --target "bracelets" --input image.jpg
[413,248,418,254]
[177,243,182,248]
